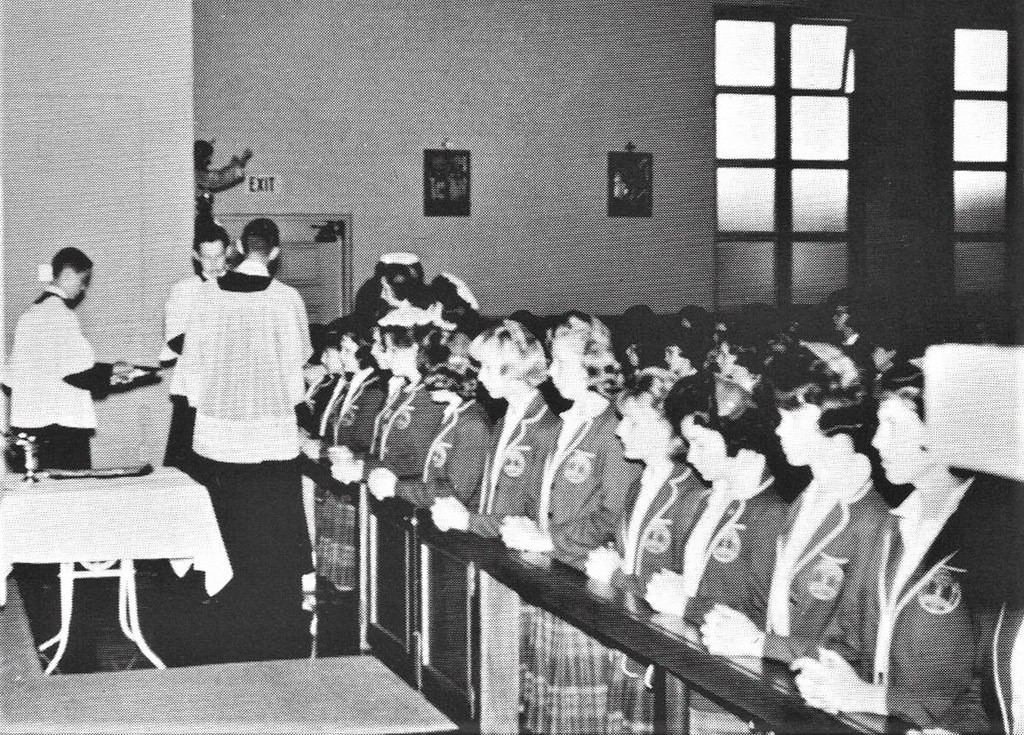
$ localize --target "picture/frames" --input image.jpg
[607,151,654,217]
[423,149,471,217]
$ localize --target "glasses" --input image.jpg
[830,309,848,317]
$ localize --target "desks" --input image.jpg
[0,466,233,676]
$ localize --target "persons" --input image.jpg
[2,247,134,470]
[191,139,253,234]
[168,217,1024,735]
